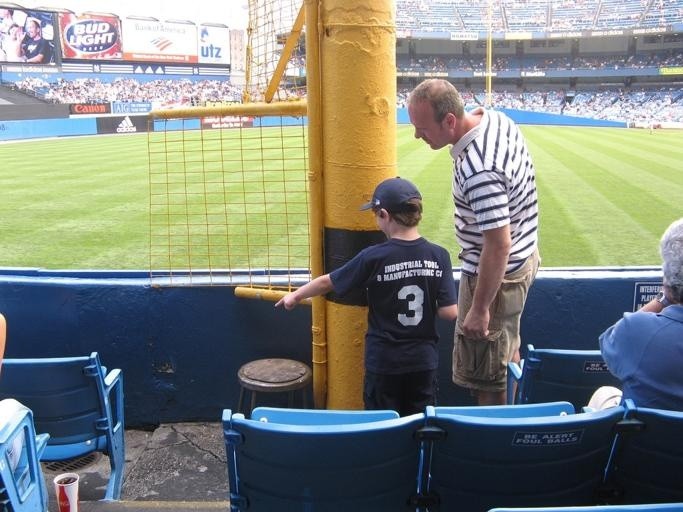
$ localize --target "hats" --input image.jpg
[359,176,422,212]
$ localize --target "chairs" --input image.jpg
[582,407,682,502]
[487,502,683,512]
[506,344,623,414]
[0,408,51,511]
[419,400,625,512]
[222,406,426,512]
[0,353,125,500]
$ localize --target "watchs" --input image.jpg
[655,291,670,307]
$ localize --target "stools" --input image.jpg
[237,358,313,419]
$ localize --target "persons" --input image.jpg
[20,75,245,107]
[274,176,458,417]
[393,2,682,128]
[1,9,52,63]
[588,219,683,419]
[408,79,541,412]
